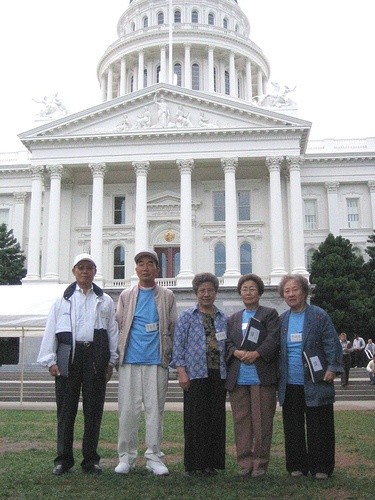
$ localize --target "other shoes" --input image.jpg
[188,467,218,476]
[252,469,266,477]
[237,468,251,475]
[355,366,357,368]
[315,473,329,480]
[362,367,365,368]
[291,471,303,476]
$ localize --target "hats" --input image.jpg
[134,249,159,265]
[73,253,97,271]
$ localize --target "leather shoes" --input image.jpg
[87,464,102,473]
[52,464,66,476]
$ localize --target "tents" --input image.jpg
[0,280,104,406]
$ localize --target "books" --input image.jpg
[347,343,352,350]
[237,316,268,353]
[303,350,329,383]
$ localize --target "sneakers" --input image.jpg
[146,462,170,475]
[115,463,134,474]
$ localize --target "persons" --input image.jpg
[168,270,236,480]
[36,254,119,476]
[224,273,281,478]
[273,275,345,481]
[336,330,375,387]
[112,249,180,476]
